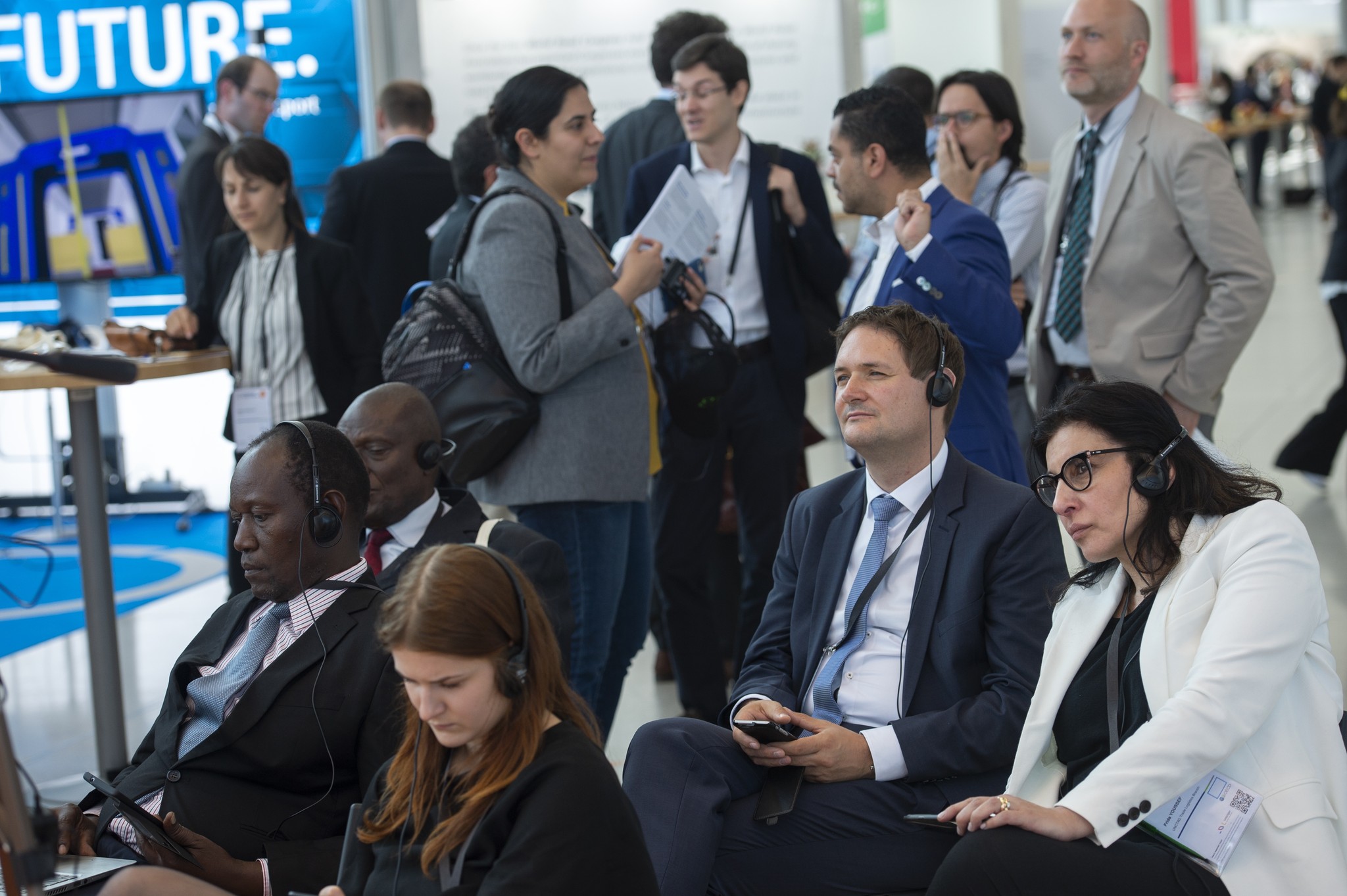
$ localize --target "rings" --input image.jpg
[996,795,1011,811]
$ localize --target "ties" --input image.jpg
[364,530,395,577]
[1051,106,1112,344]
[175,600,292,762]
[795,496,904,742]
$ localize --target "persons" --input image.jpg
[588,11,735,685]
[90,541,663,896]
[172,52,303,325]
[934,64,1053,510]
[1210,50,1347,505]
[337,381,578,694]
[844,60,937,287]
[621,36,852,737]
[460,62,708,767]
[1026,0,1274,573]
[37,416,414,896]
[920,380,1347,895]
[313,80,464,424]
[160,136,392,618]
[829,85,1040,488]
[619,304,1070,896]
[426,113,505,285]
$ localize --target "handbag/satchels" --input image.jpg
[374,186,573,486]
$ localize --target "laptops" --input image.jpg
[0,848,137,896]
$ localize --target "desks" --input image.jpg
[1208,107,1315,207]
[0,330,241,781]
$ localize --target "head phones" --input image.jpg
[1132,425,1189,498]
[416,436,457,470]
[461,542,532,701]
[274,420,340,542]
[926,316,954,407]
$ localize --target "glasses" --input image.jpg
[930,109,994,129]
[1031,445,1158,510]
[669,85,729,105]
[244,84,282,110]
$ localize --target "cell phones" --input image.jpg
[903,814,998,830]
[732,719,798,745]
[83,771,164,831]
